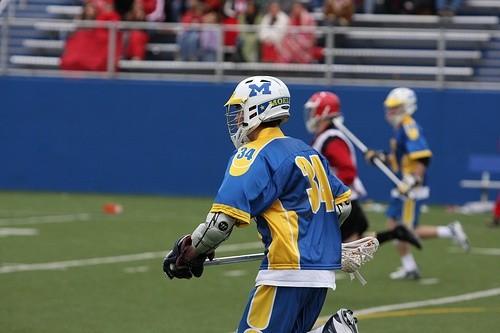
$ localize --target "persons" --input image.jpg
[60,0,467,71]
[163,76,358,333]
[303,92,422,250]
[363,88,468,280]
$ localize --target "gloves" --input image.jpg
[365,149,386,166]
[163,234,215,280]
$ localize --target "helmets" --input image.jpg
[224,75,291,150]
[304,91,340,135]
[383,87,417,128]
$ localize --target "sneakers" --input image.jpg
[390,265,420,280]
[448,220,471,253]
[322,308,359,333]
[396,224,422,249]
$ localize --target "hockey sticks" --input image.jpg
[332,117,416,200]
[169,236,380,273]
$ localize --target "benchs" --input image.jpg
[11,0,500,81]
[460,154,500,204]
